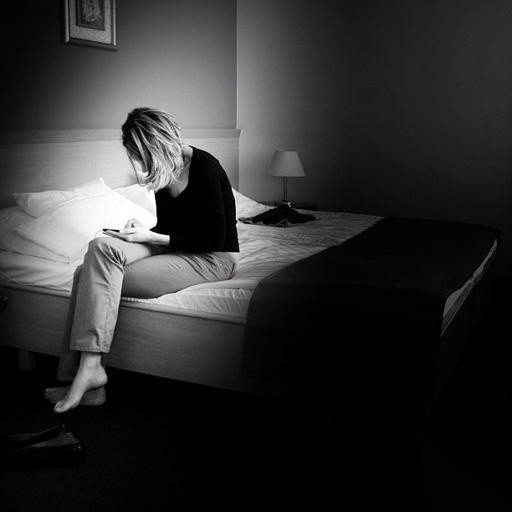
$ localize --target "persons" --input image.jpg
[42,108,240,413]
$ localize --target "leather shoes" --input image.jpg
[3,423,86,466]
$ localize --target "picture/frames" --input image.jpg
[62,0,120,55]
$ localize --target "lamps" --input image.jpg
[265,148,305,208]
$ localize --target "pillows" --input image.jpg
[1,177,273,264]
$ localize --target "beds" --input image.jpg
[1,199,510,430]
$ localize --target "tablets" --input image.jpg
[102,226,136,233]
